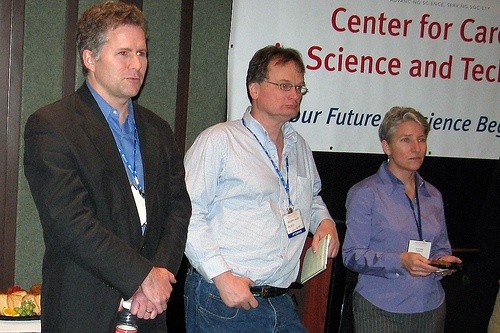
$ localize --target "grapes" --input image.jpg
[14,300,37,316]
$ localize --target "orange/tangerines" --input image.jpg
[2,307,19,316]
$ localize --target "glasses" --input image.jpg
[260,78,308,95]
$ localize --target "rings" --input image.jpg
[441,272,444,276]
[146,310,152,314]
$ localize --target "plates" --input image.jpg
[0,315,41,320]
[429,262,464,270]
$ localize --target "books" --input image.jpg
[300,233,331,283]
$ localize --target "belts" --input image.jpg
[187,263,304,299]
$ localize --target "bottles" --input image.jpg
[115,300,138,333]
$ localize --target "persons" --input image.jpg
[22,2,192,333]
[185,47,340,333]
[342,105,462,333]
